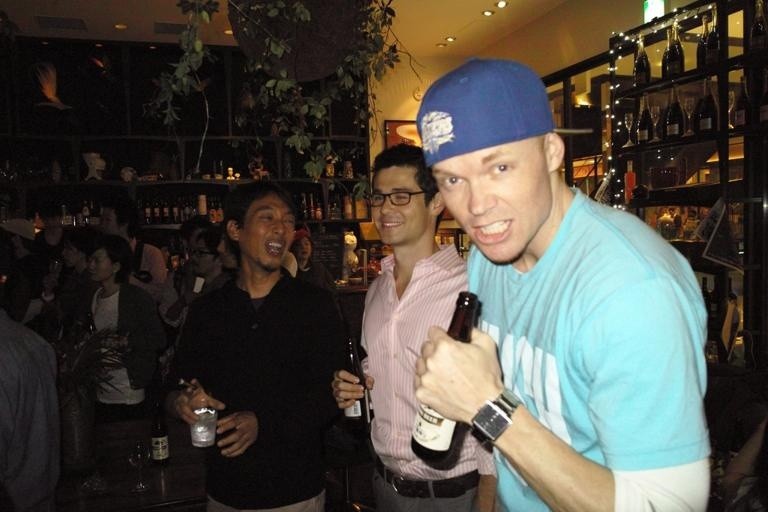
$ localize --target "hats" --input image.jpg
[416,56,594,167]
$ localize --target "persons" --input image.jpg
[720,400,767,511]
[2,312,62,512]
[1,198,330,423]
[336,142,506,512]
[415,57,719,512]
[167,182,355,511]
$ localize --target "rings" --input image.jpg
[235,415,241,424]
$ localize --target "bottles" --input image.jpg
[628,0,768,146]
[148,398,171,466]
[409,290,479,471]
[339,319,370,426]
[325,154,335,177]
[343,158,354,179]
[80,194,223,225]
[700,276,739,330]
[293,191,353,222]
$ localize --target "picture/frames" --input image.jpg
[381,118,458,222]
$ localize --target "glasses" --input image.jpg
[369,189,425,206]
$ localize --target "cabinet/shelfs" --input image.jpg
[6,35,372,231]
[541,0,765,379]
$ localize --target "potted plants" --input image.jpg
[50,304,127,469]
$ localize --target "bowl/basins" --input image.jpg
[349,278,362,284]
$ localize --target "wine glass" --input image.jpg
[126,439,150,493]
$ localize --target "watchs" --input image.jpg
[470,386,521,457]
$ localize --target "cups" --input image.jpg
[188,407,217,448]
[49,260,63,288]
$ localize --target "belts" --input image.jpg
[374,454,480,498]
